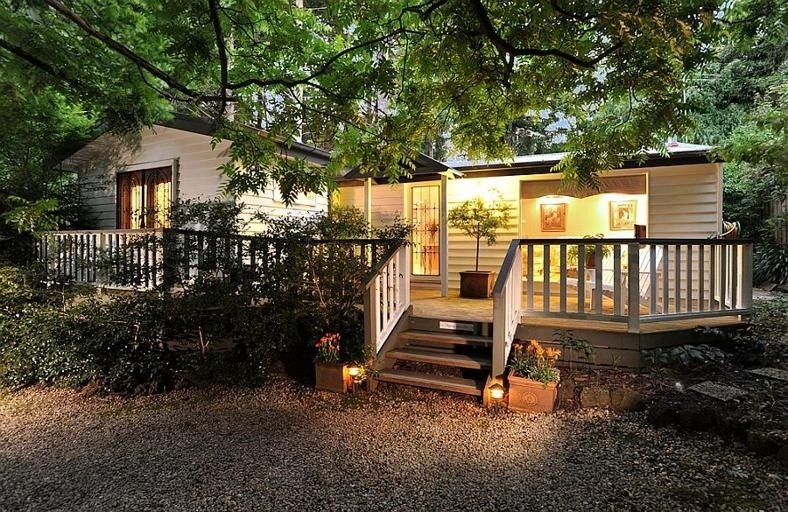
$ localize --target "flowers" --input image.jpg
[316,332,341,365]
[511,340,560,383]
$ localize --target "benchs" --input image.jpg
[561,242,665,312]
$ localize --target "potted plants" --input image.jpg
[581,234,610,268]
[447,194,513,298]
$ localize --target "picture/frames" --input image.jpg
[609,198,638,231]
[540,203,567,232]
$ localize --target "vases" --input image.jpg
[505,368,559,415]
[317,362,350,393]
